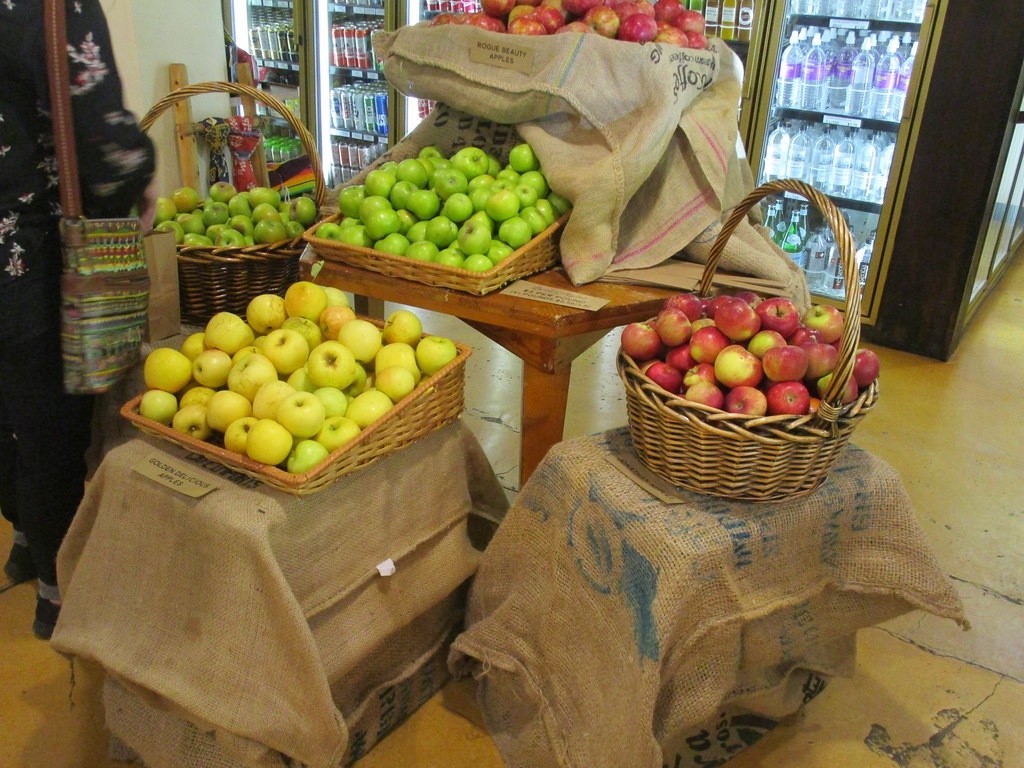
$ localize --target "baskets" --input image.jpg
[302,208,569,297]
[136,81,325,326]
[617,178,878,505]
[122,313,472,498]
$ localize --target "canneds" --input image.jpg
[247,0,388,190]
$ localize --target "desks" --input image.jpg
[301,253,770,555]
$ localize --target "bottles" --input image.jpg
[681,0,755,41]
[764,197,877,301]
[775,0,928,124]
[762,116,899,206]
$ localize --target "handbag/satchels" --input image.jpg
[143,230,181,344]
[57,216,150,396]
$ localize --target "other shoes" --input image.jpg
[4,541,39,581]
[33,594,62,639]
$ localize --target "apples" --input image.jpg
[621,292,879,417]
[429,0,709,48]
[315,143,573,274]
[139,281,456,475]
[148,181,316,248]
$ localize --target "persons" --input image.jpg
[0,0,154,638]
